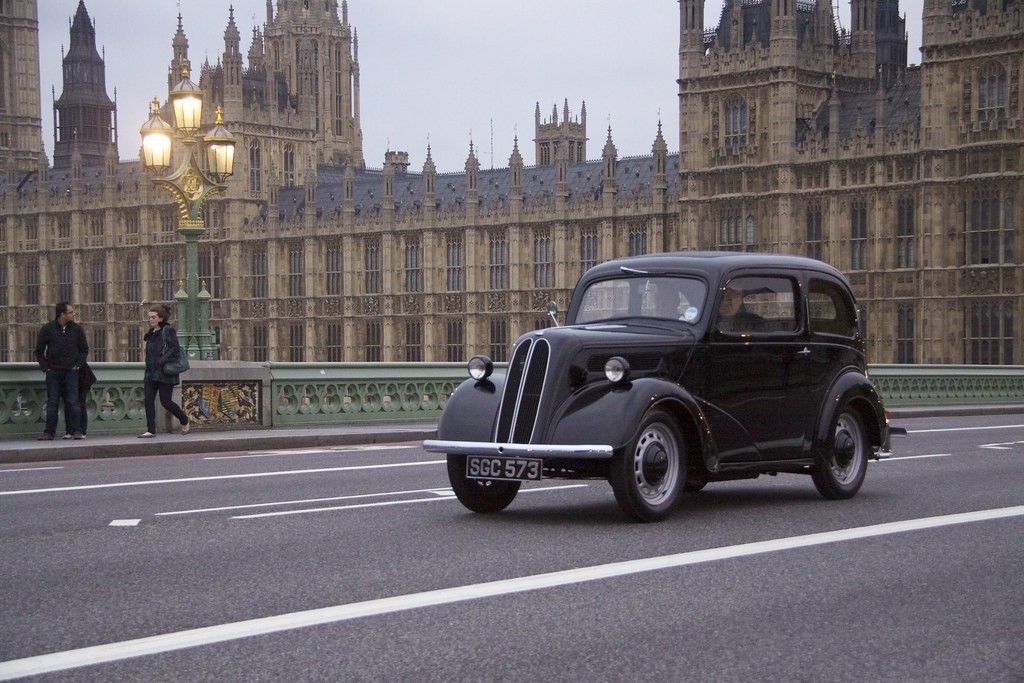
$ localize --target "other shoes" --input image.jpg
[37,433,54,440]
[137,431,156,438]
[181,416,190,435]
[63,434,71,439]
[81,434,86,439]
[73,432,82,439]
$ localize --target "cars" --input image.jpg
[424,252,905,520]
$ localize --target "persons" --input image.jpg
[137,304,189,438]
[35,301,89,439]
[715,280,772,334]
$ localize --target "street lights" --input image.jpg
[139,53,236,359]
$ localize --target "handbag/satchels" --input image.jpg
[162,326,191,376]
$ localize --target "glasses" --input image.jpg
[64,311,73,314]
